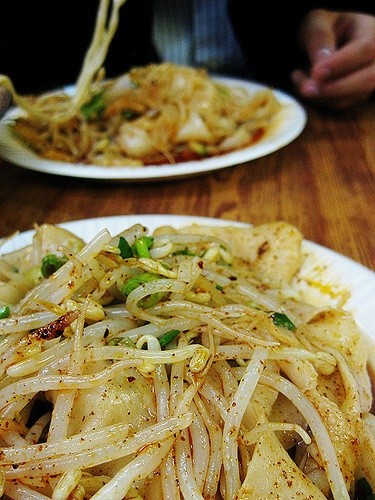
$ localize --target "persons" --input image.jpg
[0,0,375,112]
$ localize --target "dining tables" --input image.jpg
[0,105,375,272]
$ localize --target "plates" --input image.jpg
[0,216,375,345]
[0,75,306,182]
